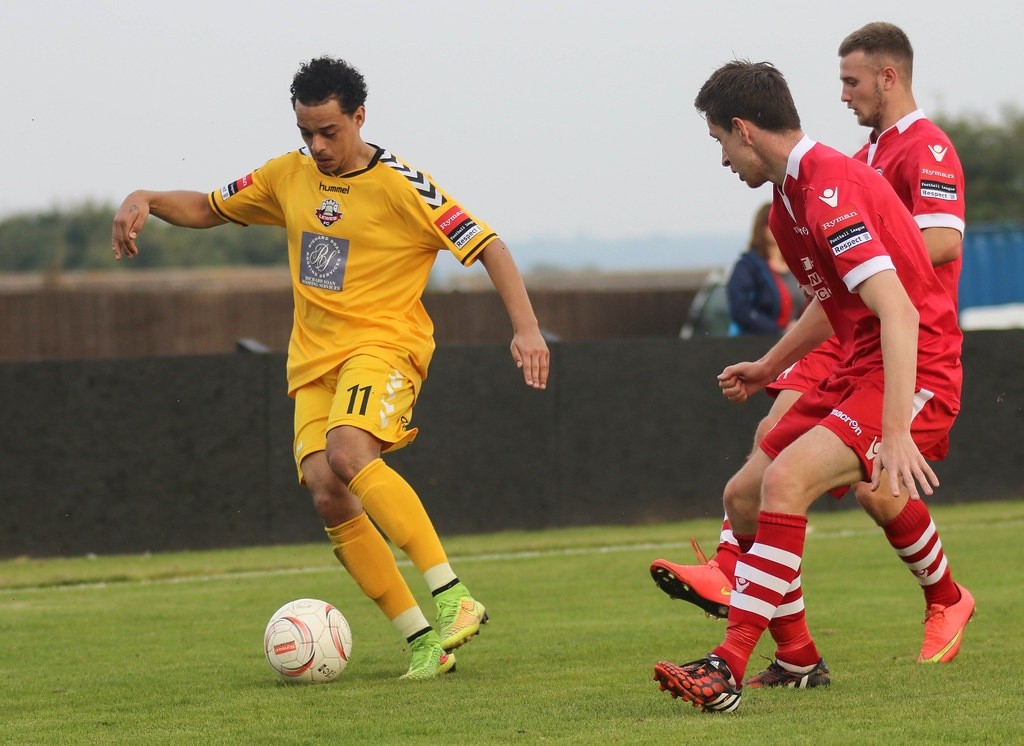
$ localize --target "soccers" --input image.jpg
[262,597,353,683]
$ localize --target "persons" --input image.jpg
[652,62,963,711]
[111,57,550,682]
[650,23,977,665]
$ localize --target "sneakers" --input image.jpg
[918,582,976,663]
[437,595,490,651]
[397,639,455,681]
[747,657,831,691]
[653,653,743,712]
[649,558,731,617]
